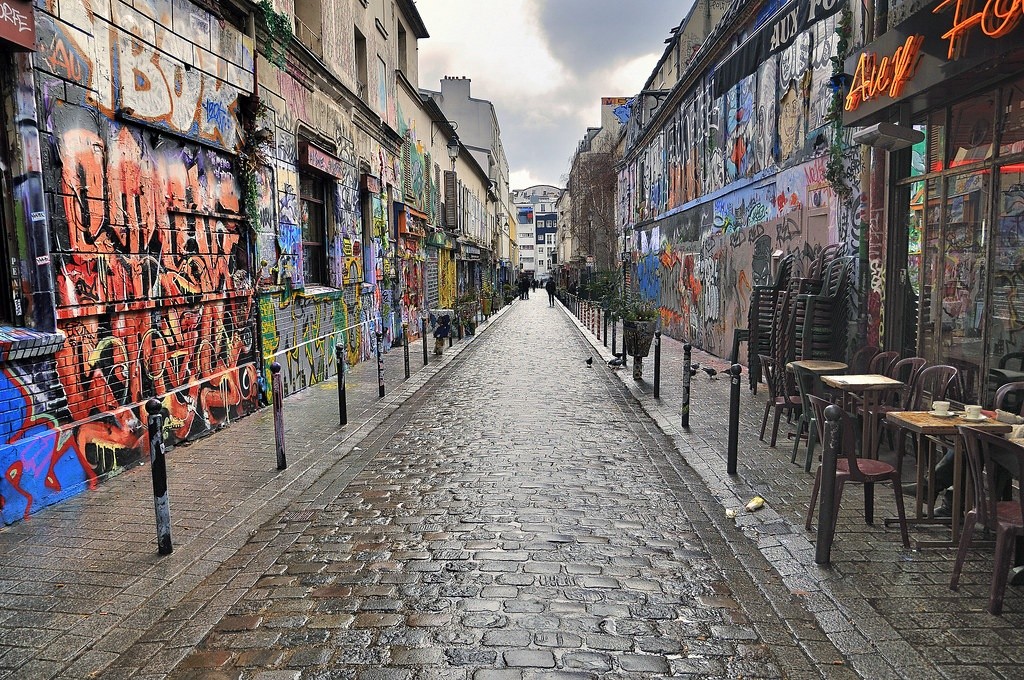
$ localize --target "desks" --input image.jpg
[820,375,904,456]
[883,411,1012,549]
[786,360,849,411]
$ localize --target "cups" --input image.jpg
[932,400,950,415]
[964,405,982,419]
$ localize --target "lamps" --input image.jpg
[851,103,925,153]
[496,215,509,230]
[430,120,460,160]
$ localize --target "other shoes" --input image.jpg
[922,506,963,517]
[889,482,939,504]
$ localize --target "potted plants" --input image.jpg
[620,302,660,357]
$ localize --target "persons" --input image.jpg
[889,432,1004,529]
[514,277,556,307]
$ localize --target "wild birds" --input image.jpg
[701,368,717,378]
[607,358,623,368]
[690,369,696,376]
[720,368,731,375]
[691,363,700,370]
[586,357,592,366]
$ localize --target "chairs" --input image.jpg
[729,243,1024,615]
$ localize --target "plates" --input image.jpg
[928,411,955,417]
[959,414,987,422]
